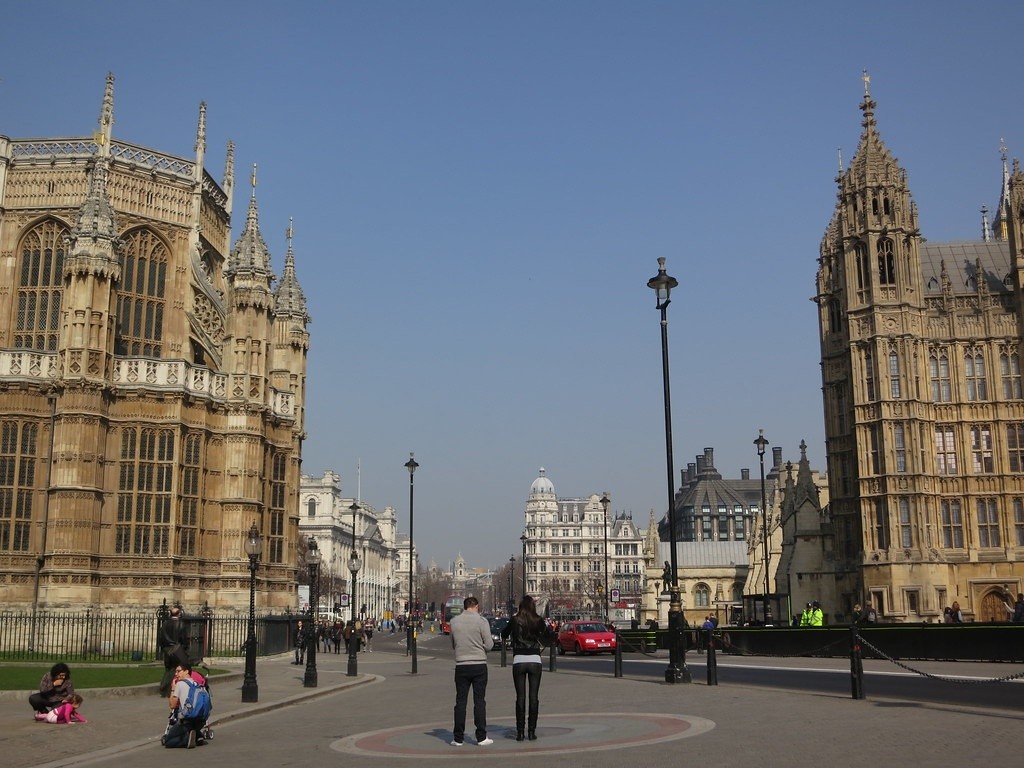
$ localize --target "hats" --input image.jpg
[807,601,813,607]
[813,600,820,607]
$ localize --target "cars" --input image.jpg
[488,615,511,651]
[558,621,616,655]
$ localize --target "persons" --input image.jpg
[311,618,374,652]
[703,613,719,630]
[1003,592,1024,621]
[944,601,962,623]
[662,561,671,591]
[500,595,554,741]
[855,599,877,624]
[34,694,87,724]
[29,662,74,714]
[449,598,494,746]
[631,617,637,629]
[390,617,424,633]
[800,600,823,627]
[159,608,189,698]
[165,665,210,748]
[544,617,567,632]
[294,621,307,666]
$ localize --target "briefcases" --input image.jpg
[167,642,189,668]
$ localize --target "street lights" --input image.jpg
[519,532,529,601]
[346,550,363,675]
[654,580,660,620]
[387,575,391,613]
[509,554,517,602]
[303,539,320,688]
[348,501,361,553]
[600,496,613,624]
[243,521,260,703]
[402,451,419,655]
[645,254,693,684]
[750,427,778,630]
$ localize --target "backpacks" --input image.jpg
[178,679,212,722]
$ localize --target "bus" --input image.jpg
[441,594,466,635]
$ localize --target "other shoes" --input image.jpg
[187,730,196,749]
[478,737,493,745]
[196,737,204,746]
[450,740,464,746]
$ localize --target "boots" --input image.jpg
[528,703,538,740]
[516,701,525,741]
